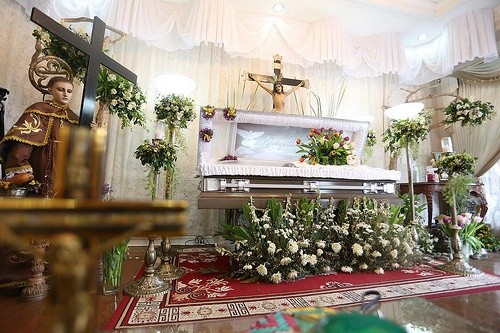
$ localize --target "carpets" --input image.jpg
[105,248,500,329]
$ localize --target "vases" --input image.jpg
[449,239,471,262]
[103,254,123,295]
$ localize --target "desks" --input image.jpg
[198,162,400,274]
[400,181,488,230]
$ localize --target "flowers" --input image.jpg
[201,103,217,120]
[32,17,196,174]
[222,106,237,120]
[438,97,497,130]
[211,193,500,284]
[104,184,131,288]
[296,126,357,166]
[429,152,479,206]
[221,155,237,162]
[365,108,434,156]
[199,127,213,142]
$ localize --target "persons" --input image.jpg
[249,73,304,113]
[2,76,96,196]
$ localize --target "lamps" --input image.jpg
[384,101,425,120]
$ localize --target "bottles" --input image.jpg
[413,167,419,181]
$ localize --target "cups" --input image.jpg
[426,169,436,182]
